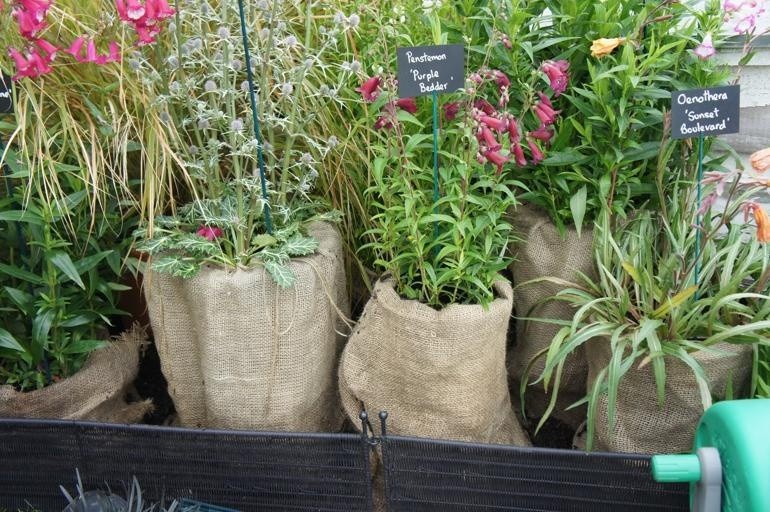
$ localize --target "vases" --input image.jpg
[135,217,359,440]
[336,269,530,451]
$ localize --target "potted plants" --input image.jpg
[476,3,721,393]
[517,176,770,462]
[0,130,143,431]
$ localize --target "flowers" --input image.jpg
[350,58,570,308]
[124,3,363,285]
[1,2,183,86]
